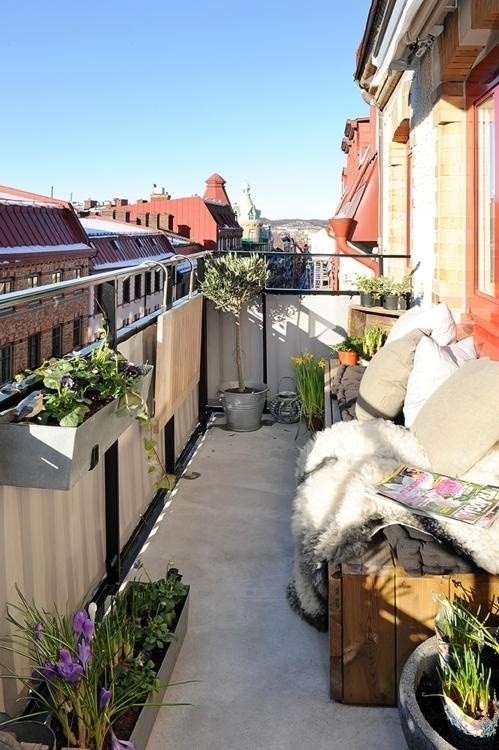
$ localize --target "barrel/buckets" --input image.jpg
[216,381,271,433]
[266,375,300,425]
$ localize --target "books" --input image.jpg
[371,465,499,525]
[473,486,499,530]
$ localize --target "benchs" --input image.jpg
[318,373,499,712]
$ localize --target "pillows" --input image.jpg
[410,357,499,477]
[446,333,477,364]
[352,326,434,423]
[381,304,434,348]
[427,299,459,349]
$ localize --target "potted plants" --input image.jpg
[357,325,385,368]
[355,276,414,312]
[331,334,359,365]
[422,593,499,679]
[195,251,273,433]
[427,645,497,737]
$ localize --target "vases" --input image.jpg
[2,361,156,492]
[395,629,497,750]
[97,574,191,750]
[302,411,323,435]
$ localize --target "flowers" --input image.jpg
[5,324,176,493]
[290,346,326,443]
[0,556,186,747]
